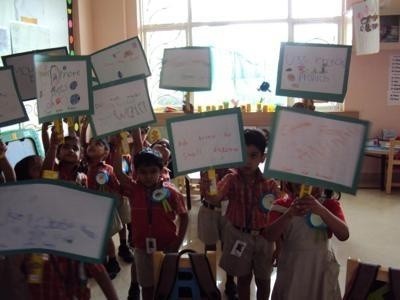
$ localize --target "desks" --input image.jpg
[361,142,399,192]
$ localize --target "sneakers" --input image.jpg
[107,257,120,280]
[118,244,134,263]
[224,281,239,300]
[127,285,141,300]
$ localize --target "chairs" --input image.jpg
[381,135,399,195]
[343,255,400,299]
[152,249,220,300]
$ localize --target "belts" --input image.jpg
[202,199,222,213]
[233,224,264,236]
